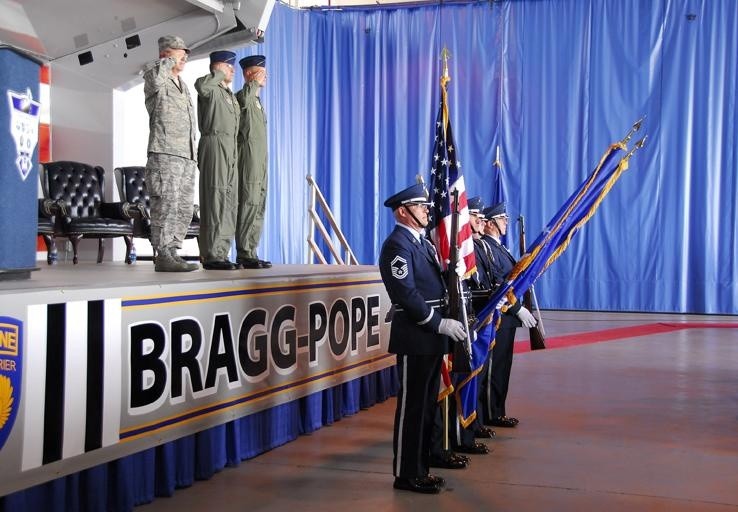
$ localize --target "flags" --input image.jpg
[425,75,485,401]
[504,160,634,316]
[448,139,629,429]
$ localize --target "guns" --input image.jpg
[517,216,546,350]
[441,190,473,372]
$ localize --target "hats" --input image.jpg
[209,52,236,65]
[467,197,508,220]
[159,35,190,54]
[384,184,432,209]
[239,55,266,68]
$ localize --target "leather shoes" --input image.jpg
[393,476,443,494]
[430,417,519,468]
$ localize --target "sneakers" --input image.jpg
[203,261,272,269]
[155,254,198,271]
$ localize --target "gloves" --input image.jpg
[437,319,468,341]
[515,306,537,329]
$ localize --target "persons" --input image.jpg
[378,184,468,493]
[140,35,198,271]
[432,197,519,470]
[194,50,243,270]
[236,55,273,270]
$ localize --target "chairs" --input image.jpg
[37,198,60,265]
[114,166,200,264]
[38,161,137,264]
